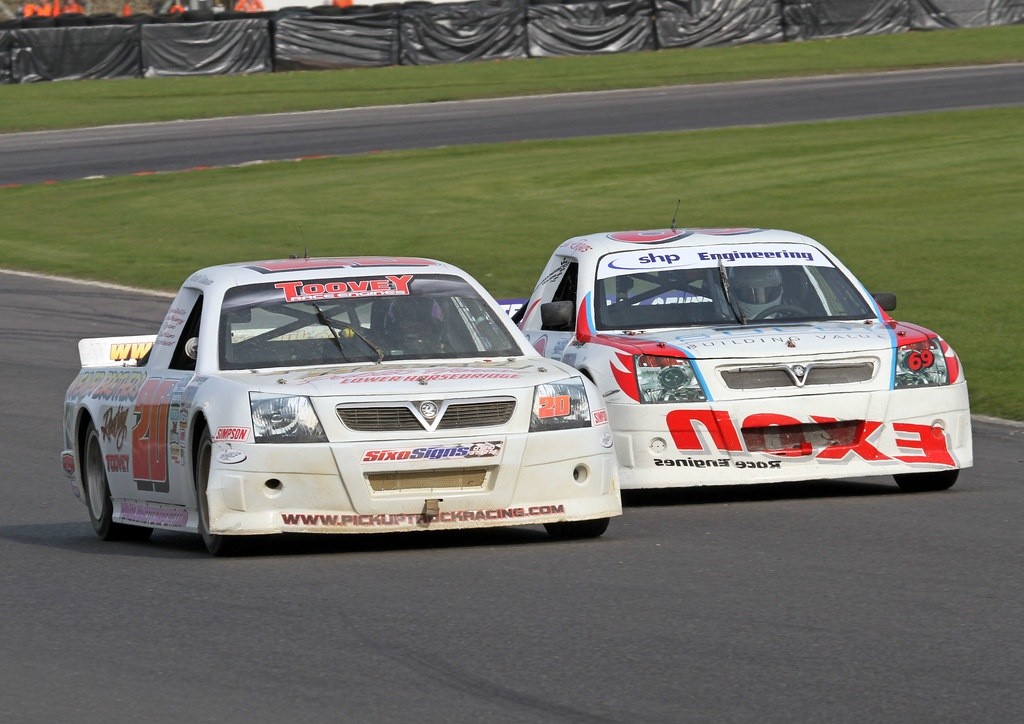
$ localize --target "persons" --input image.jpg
[384,296,446,357]
[727,265,786,324]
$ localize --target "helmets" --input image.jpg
[383,296,443,352]
[729,266,783,320]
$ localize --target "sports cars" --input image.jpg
[493,225,974,491]
[59,252,624,558]
